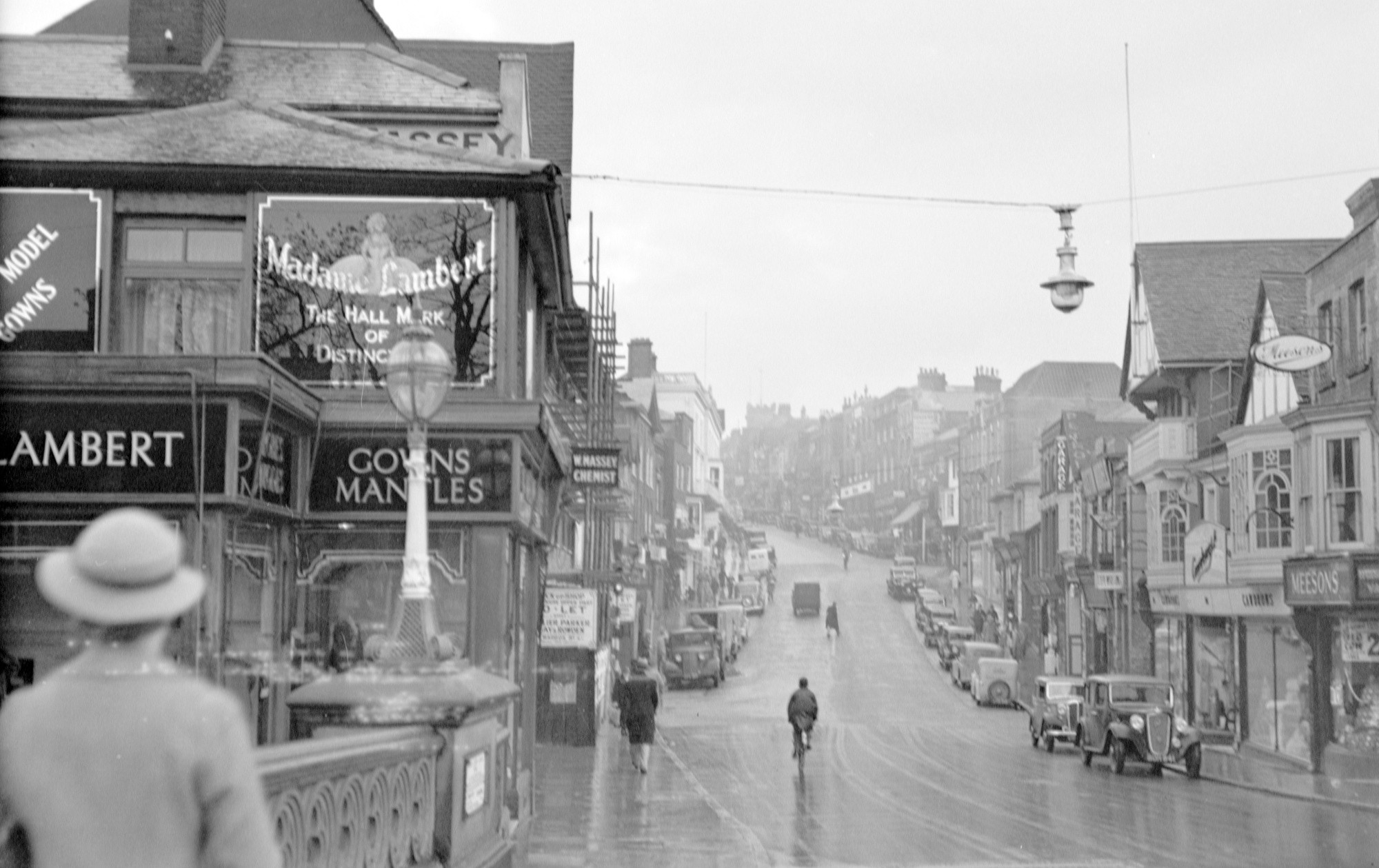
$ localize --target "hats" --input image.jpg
[632,657,649,668]
[36,507,205,624]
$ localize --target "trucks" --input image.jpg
[661,627,726,688]
[792,581,821,617]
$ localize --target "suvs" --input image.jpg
[688,527,776,662]
[1029,674,1084,753]
[1079,674,1204,779]
[887,553,1019,708]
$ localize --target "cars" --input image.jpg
[775,511,880,557]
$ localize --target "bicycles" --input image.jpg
[789,714,817,764]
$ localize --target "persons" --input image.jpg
[843,547,851,569]
[788,676,818,756]
[657,630,669,670]
[641,630,651,657]
[716,566,735,598]
[950,566,960,593]
[1,505,282,868]
[615,657,665,774]
[767,569,776,602]
[972,603,1017,649]
[826,602,839,636]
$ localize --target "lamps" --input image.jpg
[1041,202,1092,312]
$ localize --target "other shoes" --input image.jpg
[807,744,811,749]
[793,751,797,758]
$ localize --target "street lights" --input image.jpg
[371,289,473,676]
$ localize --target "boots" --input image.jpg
[640,743,650,774]
[630,743,639,768]
